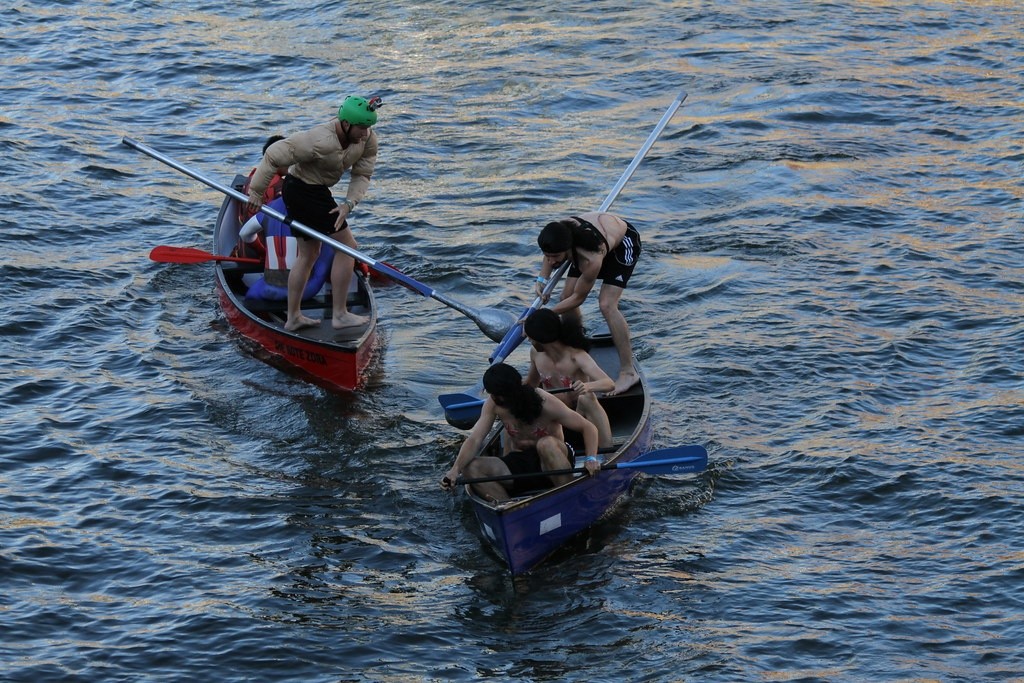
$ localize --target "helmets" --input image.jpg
[338,94,385,128]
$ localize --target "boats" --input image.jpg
[211,168,377,393]
[462,321,652,591]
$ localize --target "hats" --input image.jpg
[483,362,522,395]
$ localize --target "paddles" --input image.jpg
[444,444,708,487]
[149,245,368,278]
[357,257,401,282]
[437,380,577,421]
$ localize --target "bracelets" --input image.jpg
[537,276,548,284]
[345,200,353,212]
[584,456,597,462]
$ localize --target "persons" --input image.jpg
[536,213,642,395]
[239,196,333,301]
[439,363,601,502]
[247,94,383,331]
[239,135,288,227]
[522,308,616,448]
